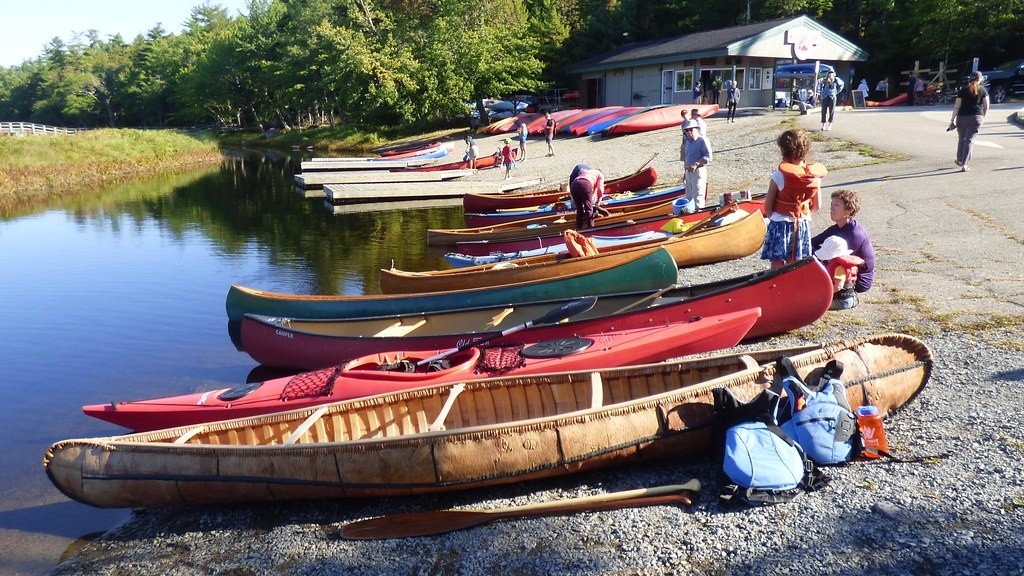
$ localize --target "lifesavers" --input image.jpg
[564,227,597,258]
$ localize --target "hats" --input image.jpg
[691,109,701,116]
[813,235,854,261]
[683,120,700,129]
[504,138,511,144]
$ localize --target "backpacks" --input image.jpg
[709,387,814,502]
[773,356,861,465]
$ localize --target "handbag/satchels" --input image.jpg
[508,158,517,170]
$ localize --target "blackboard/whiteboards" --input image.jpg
[851,90,866,108]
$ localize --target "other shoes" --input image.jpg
[826,127,830,131]
[955,158,970,172]
[821,127,827,131]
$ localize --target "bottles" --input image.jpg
[858,407,889,458]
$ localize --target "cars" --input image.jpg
[962,58,1024,104]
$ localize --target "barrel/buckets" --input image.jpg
[673,198,688,214]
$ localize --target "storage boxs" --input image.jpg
[719,190,752,208]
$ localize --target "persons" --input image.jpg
[760,130,874,310]
[908,72,923,104]
[681,109,713,209]
[569,164,605,229]
[799,71,844,131]
[857,78,889,101]
[693,76,740,123]
[464,113,555,180]
[950,72,989,172]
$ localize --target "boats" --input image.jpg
[80,305,765,435]
[292,90,770,269]
[39,330,938,512]
[225,244,679,359]
[240,254,836,373]
[380,207,769,297]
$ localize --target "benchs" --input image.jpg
[373,319,426,337]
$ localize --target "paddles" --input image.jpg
[414,294,599,368]
[677,199,739,238]
[338,477,704,542]
[611,283,676,314]
